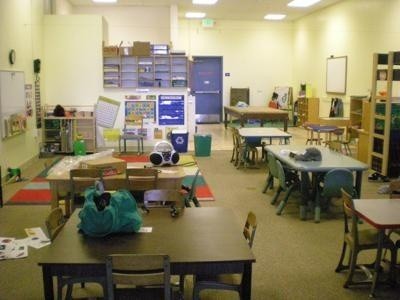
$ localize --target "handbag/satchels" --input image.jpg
[78,180,141,237]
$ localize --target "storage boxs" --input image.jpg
[102,40,151,56]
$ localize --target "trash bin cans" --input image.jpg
[194,133,212,157]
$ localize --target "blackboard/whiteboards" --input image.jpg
[0,70,28,140]
[325,56,348,95]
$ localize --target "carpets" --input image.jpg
[3,154,215,206]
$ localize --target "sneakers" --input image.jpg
[368,172,389,182]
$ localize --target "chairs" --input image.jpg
[223,125,399,299]
[46,167,258,300]
[118,114,145,156]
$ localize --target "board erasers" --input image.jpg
[330,55,334,57]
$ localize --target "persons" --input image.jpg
[268,92,279,110]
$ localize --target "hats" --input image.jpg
[296,146,322,160]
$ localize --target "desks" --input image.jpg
[224,105,289,132]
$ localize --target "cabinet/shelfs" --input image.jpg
[297,96,319,126]
[40,104,98,155]
[103,55,189,87]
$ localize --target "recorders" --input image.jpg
[149,141,179,166]
